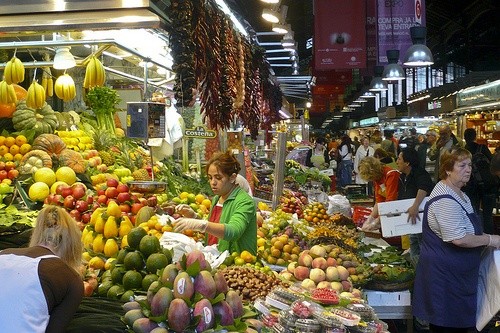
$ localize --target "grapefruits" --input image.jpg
[27,167,76,201]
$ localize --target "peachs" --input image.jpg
[279,243,361,299]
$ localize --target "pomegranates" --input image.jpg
[0,160,19,180]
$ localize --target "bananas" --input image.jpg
[83,55,105,88]
[0,56,77,109]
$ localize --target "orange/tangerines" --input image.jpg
[205,120,243,160]
[0,135,32,161]
[153,191,299,264]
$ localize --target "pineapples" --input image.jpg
[94,132,159,182]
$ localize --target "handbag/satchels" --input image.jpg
[334,149,342,161]
[327,194,354,218]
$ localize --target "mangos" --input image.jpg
[78,200,245,333]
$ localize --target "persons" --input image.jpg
[465,128,500,235]
[308,127,436,196]
[0,205,98,333]
[358,156,402,246]
[175,148,257,257]
[395,146,434,257]
[412,145,500,333]
[428,124,463,184]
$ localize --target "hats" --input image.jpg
[411,127,417,132]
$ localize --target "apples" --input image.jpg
[55,129,132,187]
[280,200,331,224]
[44,180,175,225]
[0,179,16,215]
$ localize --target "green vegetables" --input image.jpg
[253,156,332,186]
[357,245,418,280]
[84,87,121,131]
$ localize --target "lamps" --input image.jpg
[354,84,368,102]
[403,27,434,65]
[276,75,318,102]
[358,76,376,98]
[381,50,405,81]
[53,34,77,70]
[369,65,388,91]
[255,0,300,76]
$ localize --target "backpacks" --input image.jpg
[471,143,493,186]
[411,139,423,160]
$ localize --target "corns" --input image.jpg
[47,78,54,96]
[41,79,47,94]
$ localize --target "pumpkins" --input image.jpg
[12,99,86,177]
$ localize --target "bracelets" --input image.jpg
[488,235,492,246]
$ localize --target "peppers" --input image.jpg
[163,0,284,139]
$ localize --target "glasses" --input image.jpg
[426,134,430,137]
